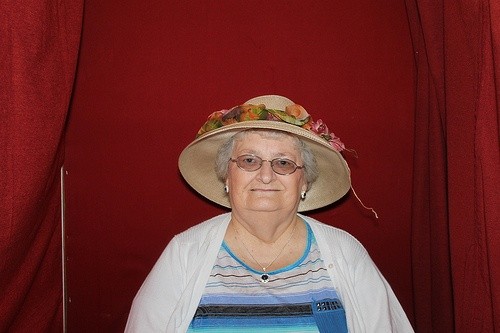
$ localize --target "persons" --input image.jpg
[122,96,414,333]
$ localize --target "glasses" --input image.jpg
[229,154,304,175]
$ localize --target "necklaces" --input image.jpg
[231,214,298,273]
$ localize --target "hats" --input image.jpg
[178,94,353,212]
